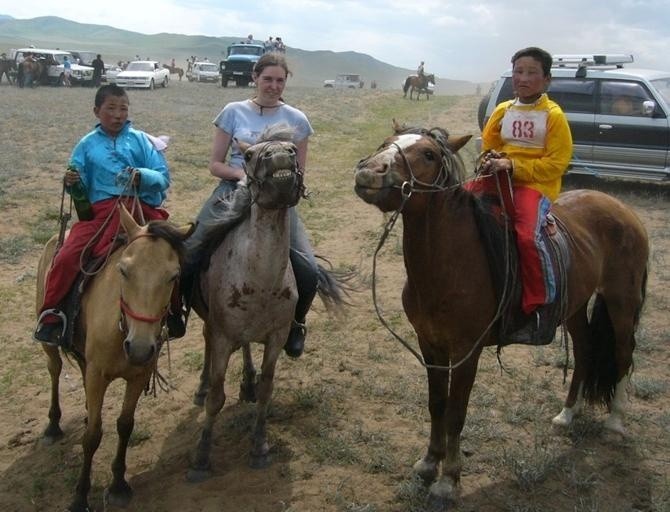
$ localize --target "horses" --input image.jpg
[162,64,183,81]
[35,198,191,512]
[353,117,651,512]
[184,121,380,482]
[0,59,16,85]
[403,73,435,101]
[19,61,43,88]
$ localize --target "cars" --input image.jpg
[117,60,170,91]
[103,64,121,86]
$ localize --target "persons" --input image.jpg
[167,50,319,360]
[34,85,186,345]
[465,46,572,344]
[611,87,643,116]
[0,35,287,91]
[417,61,426,80]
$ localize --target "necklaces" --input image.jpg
[253,99,286,117]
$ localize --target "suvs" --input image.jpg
[324,73,364,91]
[11,48,94,88]
[67,50,110,82]
[477,52,670,186]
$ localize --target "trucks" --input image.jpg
[219,44,273,87]
[187,62,219,83]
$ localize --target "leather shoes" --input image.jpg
[287,322,305,358]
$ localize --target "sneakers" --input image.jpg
[507,316,548,343]
[166,313,186,338]
[33,319,62,342]
